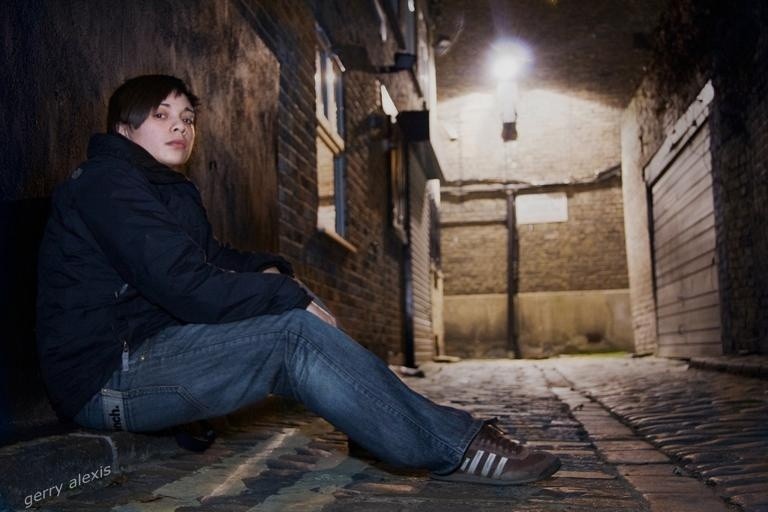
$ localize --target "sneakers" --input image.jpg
[429,422,562,485]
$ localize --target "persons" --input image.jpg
[35,74,563,485]
